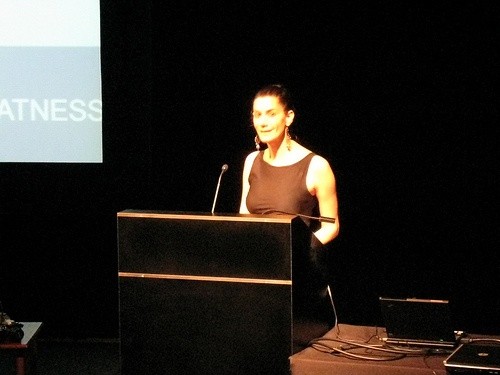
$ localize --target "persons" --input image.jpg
[239,82,341,245]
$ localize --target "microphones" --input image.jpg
[210,164,228,216]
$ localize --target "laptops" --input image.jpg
[379,297,464,348]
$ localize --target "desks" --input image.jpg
[0,322,42,375]
[287,324,500,374]
